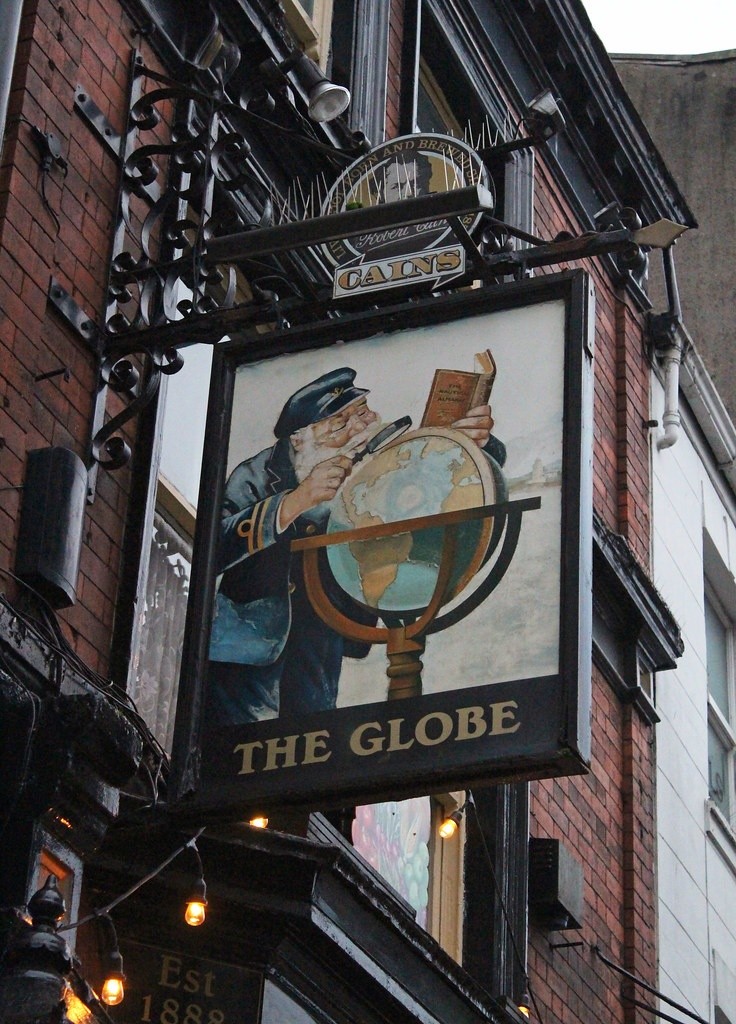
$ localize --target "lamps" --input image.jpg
[279,45,351,121]
[594,201,627,231]
[477,89,567,158]
